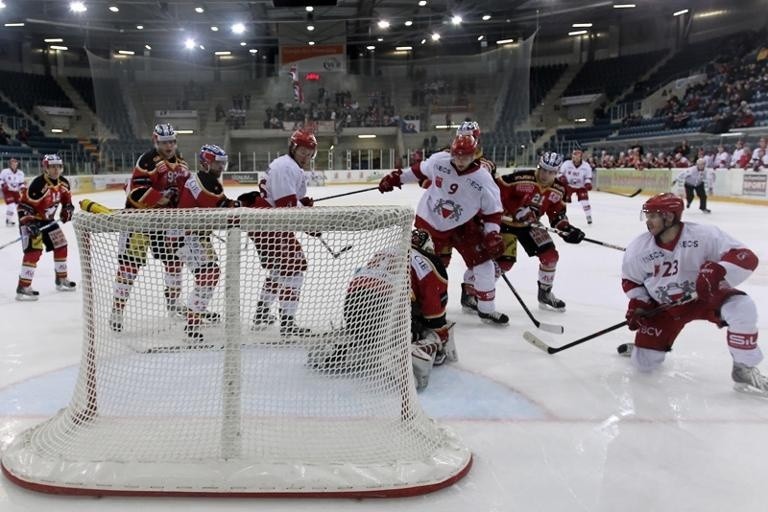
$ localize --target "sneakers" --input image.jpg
[461,284,508,323]
[166,303,220,341]
[587,216,591,224]
[5,219,15,224]
[281,316,310,338]
[255,302,276,324]
[732,363,767,391]
[110,308,124,333]
[538,281,565,308]
[56,278,76,286]
[17,283,39,295]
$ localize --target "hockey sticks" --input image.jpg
[318,235,351,257]
[592,188,642,197]
[124,337,308,354]
[213,232,250,252]
[492,259,564,334]
[523,292,698,354]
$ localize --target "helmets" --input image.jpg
[287,130,317,154]
[535,152,562,186]
[152,123,177,148]
[199,143,228,171]
[411,228,436,255]
[451,122,483,163]
[42,154,64,176]
[640,193,683,223]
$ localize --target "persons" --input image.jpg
[671,158,711,212]
[418,121,501,310]
[163,144,244,340]
[0,158,25,226]
[309,229,449,390]
[617,192,767,391]
[378,135,509,324]
[215,64,475,147]
[243,128,321,337]
[109,123,191,332]
[585,38,768,171]
[556,149,592,224]
[17,154,76,296]
[495,152,585,307]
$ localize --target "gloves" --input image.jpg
[25,221,40,238]
[60,208,73,223]
[560,176,567,185]
[557,220,585,243]
[379,168,403,192]
[696,261,726,301]
[484,233,505,257]
[626,298,657,330]
[585,183,592,190]
